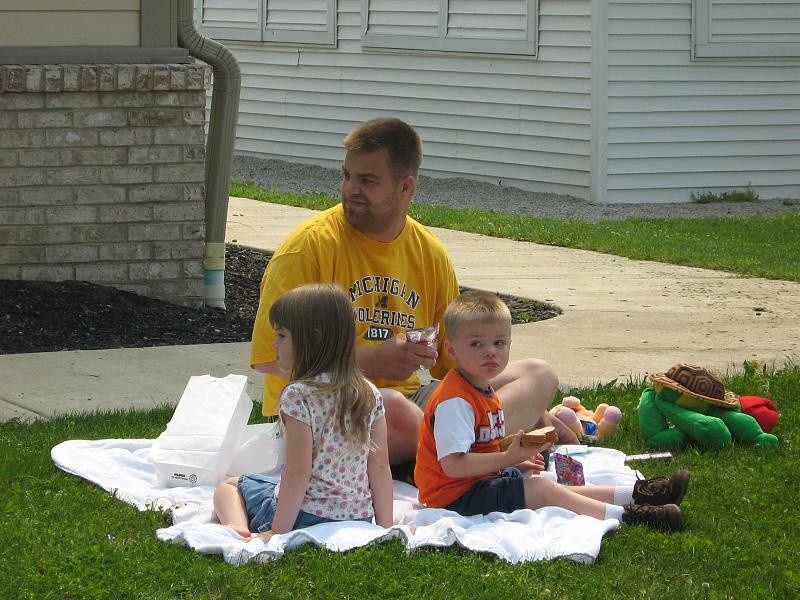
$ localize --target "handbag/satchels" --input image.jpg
[148,371,253,487]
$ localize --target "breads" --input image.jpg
[498,426,556,451]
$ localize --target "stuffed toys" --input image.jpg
[636,363,780,449]
[546,396,622,443]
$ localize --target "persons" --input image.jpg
[414,290,691,533]
[213,283,395,545]
[249,117,560,468]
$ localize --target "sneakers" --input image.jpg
[621,501,682,532]
[632,471,690,506]
